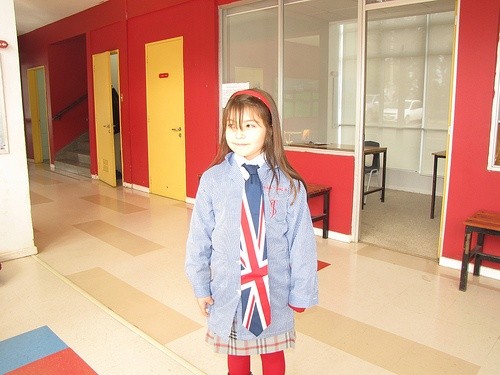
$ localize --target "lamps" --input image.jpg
[283,128,310,144]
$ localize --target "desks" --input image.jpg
[282,142,387,211]
[430,150,446,219]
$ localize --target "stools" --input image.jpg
[304,181,332,239]
[458,210,500,292]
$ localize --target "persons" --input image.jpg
[185,88,319,375]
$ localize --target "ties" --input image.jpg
[240,163,271,337]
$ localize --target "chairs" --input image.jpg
[364,141,382,206]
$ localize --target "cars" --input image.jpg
[384,99,422,124]
[366,94,392,119]
